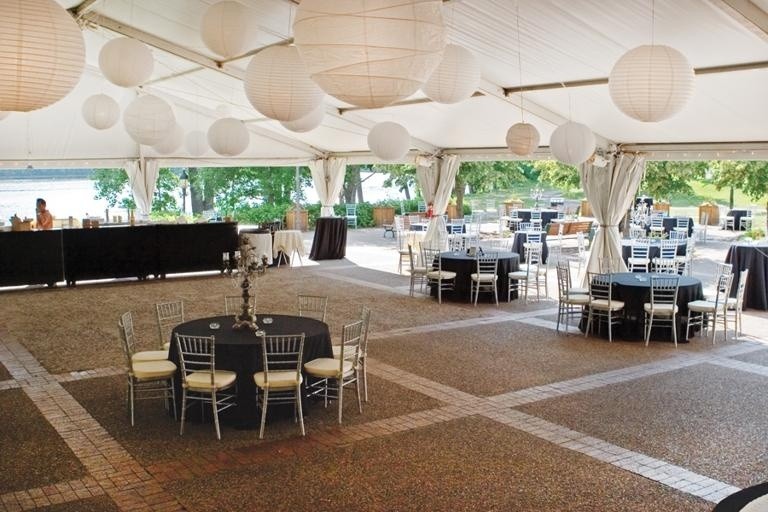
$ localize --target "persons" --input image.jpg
[426,202,434,218]
[36,198,53,229]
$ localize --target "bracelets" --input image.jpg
[37,212,41,216]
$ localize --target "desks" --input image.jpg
[0,230,64,289]
[63,221,242,289]
[724,240,767,310]
[273,230,304,269]
[309,217,348,260]
[242,234,273,264]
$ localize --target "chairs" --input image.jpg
[174,332,239,440]
[346,203,358,228]
[304,320,362,424]
[224,293,256,315]
[332,305,372,402]
[156,300,185,350]
[118,320,177,427]
[121,310,170,409]
[254,332,306,439]
[297,294,328,322]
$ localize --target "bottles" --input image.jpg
[216,209,222,223]
